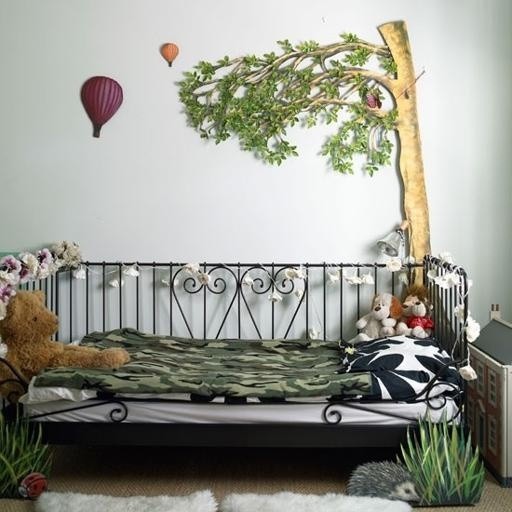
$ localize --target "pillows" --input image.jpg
[339,338,454,372]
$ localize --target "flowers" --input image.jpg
[0,242,83,322]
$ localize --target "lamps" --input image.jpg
[379,222,409,257]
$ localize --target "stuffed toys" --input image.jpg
[393,280,437,339]
[347,291,401,347]
[1,289,133,402]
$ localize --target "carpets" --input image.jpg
[219,491,414,511]
[32,491,218,512]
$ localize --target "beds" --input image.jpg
[0,240,469,456]
